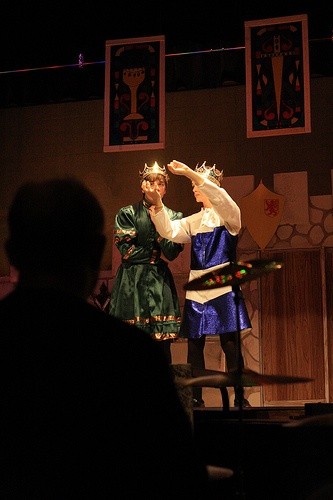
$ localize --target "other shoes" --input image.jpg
[192,400,205,408]
[234,398,252,408]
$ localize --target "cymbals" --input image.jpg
[176,368,315,388]
[182,258,284,291]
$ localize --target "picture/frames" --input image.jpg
[245,14,311,139]
[103,35,166,152]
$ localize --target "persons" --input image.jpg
[0,178,234,500]
[108,160,183,342]
[142,159,252,408]
